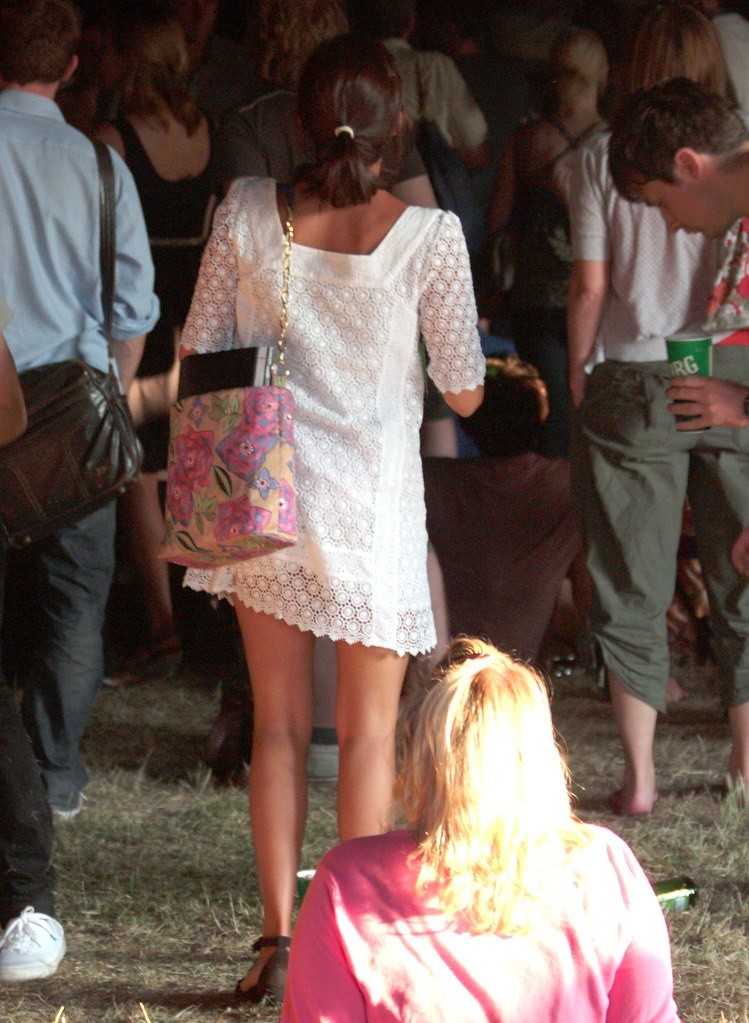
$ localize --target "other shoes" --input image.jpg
[609,789,627,816]
[0,906,68,983]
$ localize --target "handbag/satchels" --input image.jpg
[0,360,144,562]
[158,182,295,570]
[416,55,490,255]
[471,129,580,317]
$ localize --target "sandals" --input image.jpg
[234,935,291,1007]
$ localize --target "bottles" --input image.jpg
[654,877,698,911]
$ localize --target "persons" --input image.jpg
[282,639,681,1023]
[606,78,748,579]
[179,40,486,1007]
[371,0,749,816]
[0,0,437,983]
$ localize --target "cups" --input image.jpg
[296,869,314,898]
[667,334,714,432]
